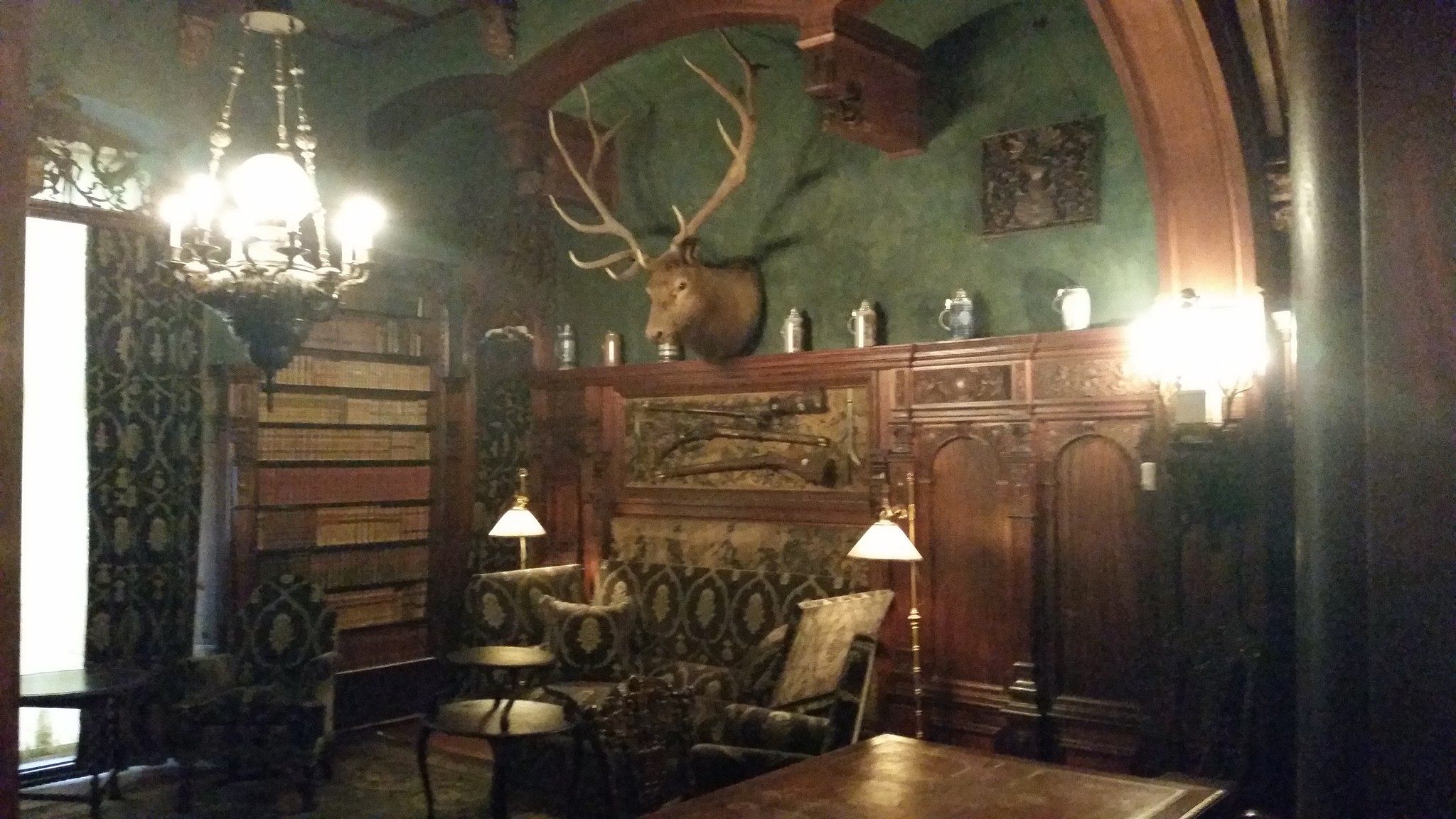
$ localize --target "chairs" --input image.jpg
[171,570,342,819]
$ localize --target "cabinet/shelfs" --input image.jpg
[227,303,447,740]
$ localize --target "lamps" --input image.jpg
[488,468,548,570]
[1120,278,1278,427]
[845,472,927,741]
[157,0,390,410]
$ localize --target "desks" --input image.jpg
[415,647,585,819]
[636,731,1233,819]
[17,666,162,819]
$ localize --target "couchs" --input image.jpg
[679,630,877,799]
[462,556,851,728]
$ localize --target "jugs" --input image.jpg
[659,341,684,363]
[848,300,877,348]
[1051,287,1092,331]
[780,308,806,352]
[938,288,975,339]
[600,330,623,366]
[554,324,578,372]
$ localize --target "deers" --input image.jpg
[546,29,771,366]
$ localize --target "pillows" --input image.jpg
[725,615,802,708]
[530,591,639,684]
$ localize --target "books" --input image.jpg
[255,312,431,634]
